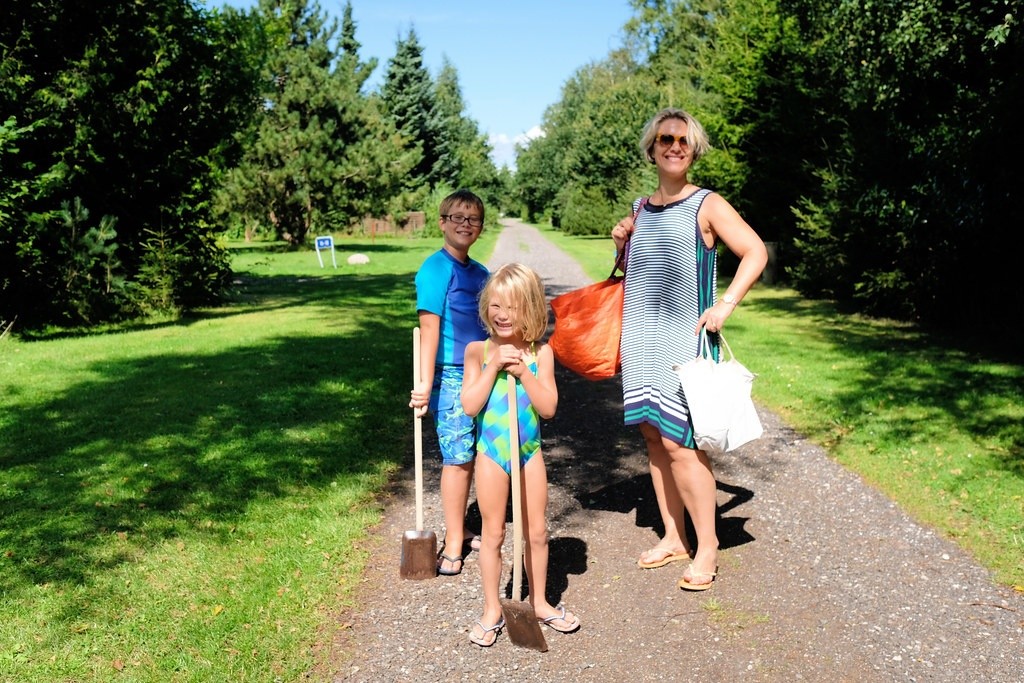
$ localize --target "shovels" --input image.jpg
[401,327,437,580]
[501,370,547,652]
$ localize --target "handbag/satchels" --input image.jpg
[672,324,762,453]
[547,275,625,381]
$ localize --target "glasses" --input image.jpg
[655,134,687,146]
[441,214,484,227]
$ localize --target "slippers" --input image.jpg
[469,619,505,645]
[462,535,481,552]
[538,607,580,631]
[436,553,462,573]
[680,564,716,589]
[638,549,693,567]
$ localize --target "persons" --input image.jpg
[407,189,505,579]
[610,106,768,595]
[459,261,580,647]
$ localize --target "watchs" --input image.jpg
[718,293,739,309]
